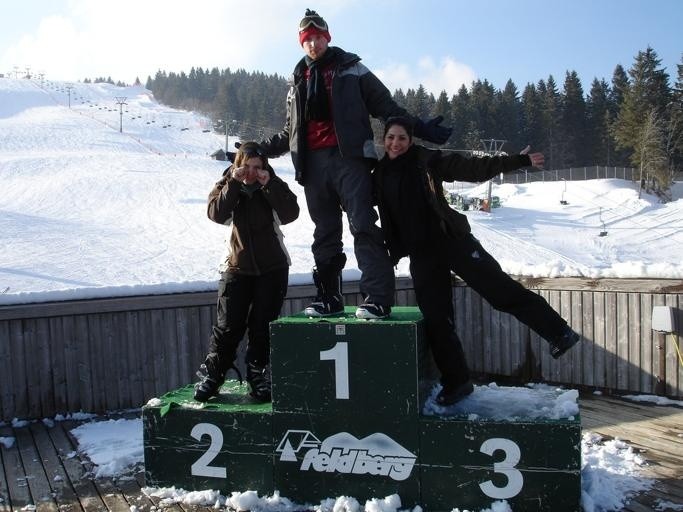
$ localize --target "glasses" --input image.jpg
[299,16,328,31]
[242,147,265,156]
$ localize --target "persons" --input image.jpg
[338,118,581,406]
[192,141,299,405]
[223,6,454,320]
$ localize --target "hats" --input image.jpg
[299,24,331,46]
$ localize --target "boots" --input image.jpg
[194,375,223,402]
[246,368,271,401]
[548,327,581,359]
[355,294,392,319]
[436,376,474,406]
[305,293,345,316]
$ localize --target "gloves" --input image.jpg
[413,115,453,145]
[226,142,241,164]
